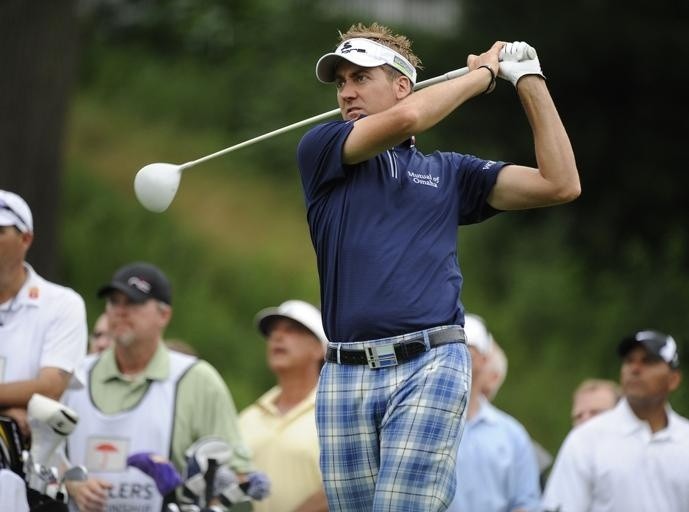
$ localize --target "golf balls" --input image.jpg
[0,392,269,512]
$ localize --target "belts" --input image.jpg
[328,327,466,371]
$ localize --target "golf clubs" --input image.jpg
[134,47,538,213]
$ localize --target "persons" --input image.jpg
[295,18,584,512]
[444,314,687,512]
[1,189,330,512]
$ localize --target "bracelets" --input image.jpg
[477,63,496,97]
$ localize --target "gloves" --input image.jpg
[495,40,548,91]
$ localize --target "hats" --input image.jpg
[314,38,418,88]
[255,298,328,360]
[1,188,34,235]
[618,328,680,370]
[97,263,170,304]
